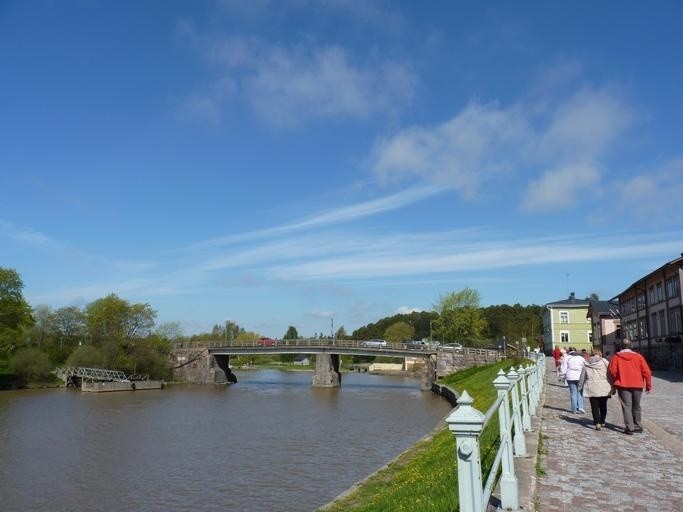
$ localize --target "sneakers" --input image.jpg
[621,426,643,434]
[594,423,605,430]
[572,408,585,414]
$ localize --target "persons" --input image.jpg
[559,346,586,416]
[561,346,589,360]
[608,339,651,434]
[603,350,611,360]
[553,345,563,372]
[577,349,616,432]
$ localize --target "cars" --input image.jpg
[401,341,462,353]
[256,337,281,347]
[360,338,387,349]
